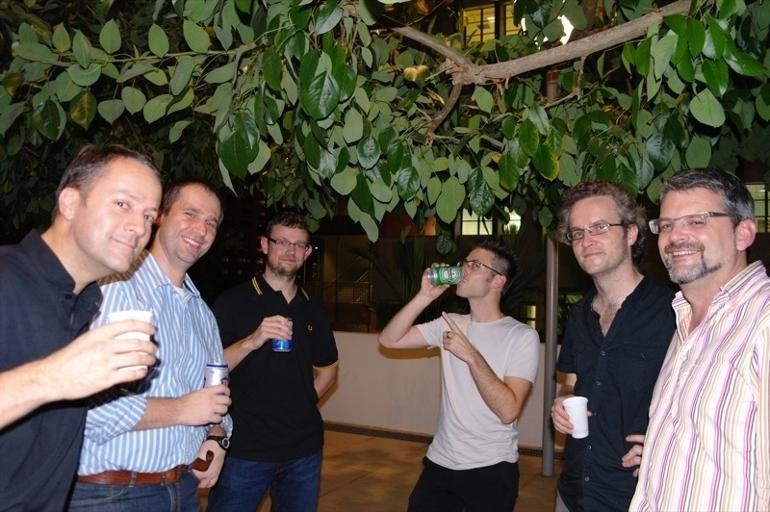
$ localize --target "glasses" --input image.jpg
[456,260,503,275]
[649,211,731,234]
[566,222,631,242]
[268,236,308,250]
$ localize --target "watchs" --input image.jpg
[205,433,231,451]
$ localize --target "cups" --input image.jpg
[561,396,590,441]
[106,310,153,347]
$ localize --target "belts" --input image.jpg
[76,462,189,487]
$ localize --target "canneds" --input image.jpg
[426,266,463,286]
[202,362,228,395]
[273,318,293,352]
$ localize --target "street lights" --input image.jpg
[519,15,575,478]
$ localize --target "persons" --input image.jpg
[547,179,678,511]
[206,210,341,511]
[628,165,769,512]
[64,180,234,512]
[376,240,539,512]
[2,143,163,512]
